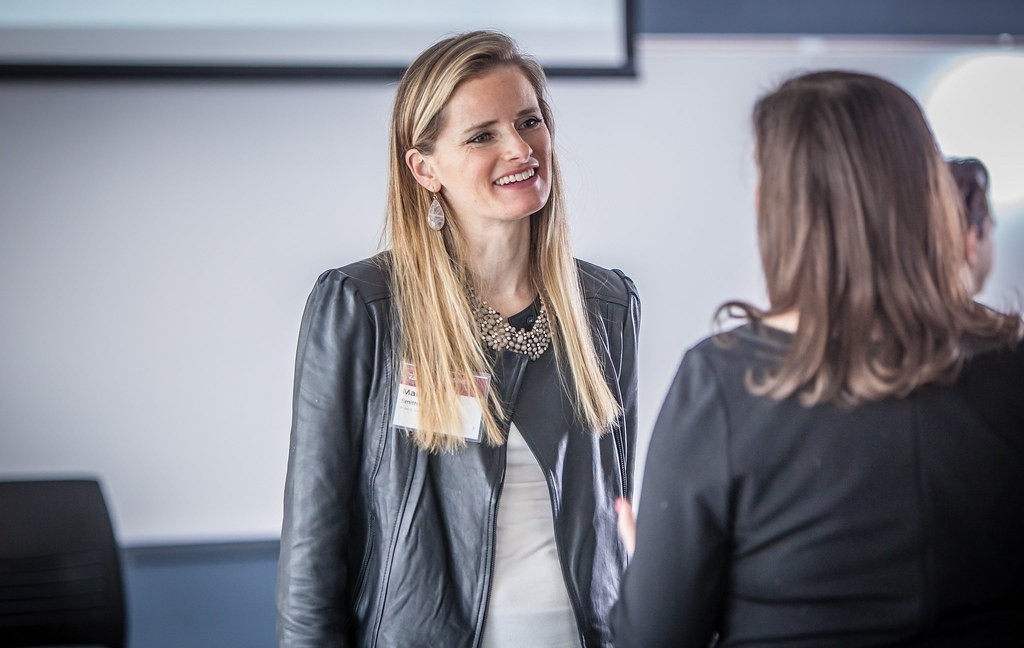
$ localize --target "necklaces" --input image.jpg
[460,266,555,360]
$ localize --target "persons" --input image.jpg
[274,28,642,647]
[944,155,998,295]
[602,67,1023,647]
